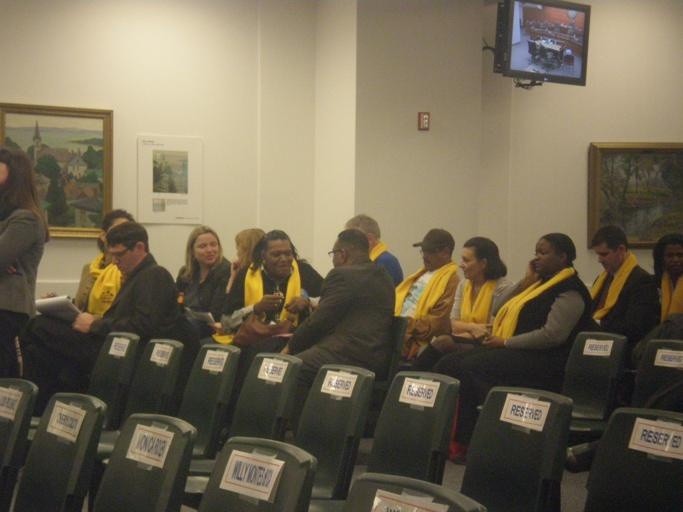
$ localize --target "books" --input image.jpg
[35,296,81,317]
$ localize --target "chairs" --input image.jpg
[1,315,682,510]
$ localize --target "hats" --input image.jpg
[412,229,454,252]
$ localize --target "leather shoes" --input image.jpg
[565,442,592,473]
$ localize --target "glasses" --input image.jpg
[328,250,340,257]
[108,246,130,258]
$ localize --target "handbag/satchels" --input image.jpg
[232,311,293,347]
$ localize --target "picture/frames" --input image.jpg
[585,140,682,251]
[0,103,115,240]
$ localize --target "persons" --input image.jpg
[0,146,50,378]
[20,220,176,417]
[41,210,133,317]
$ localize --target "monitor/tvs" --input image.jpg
[493,0,590,86]
